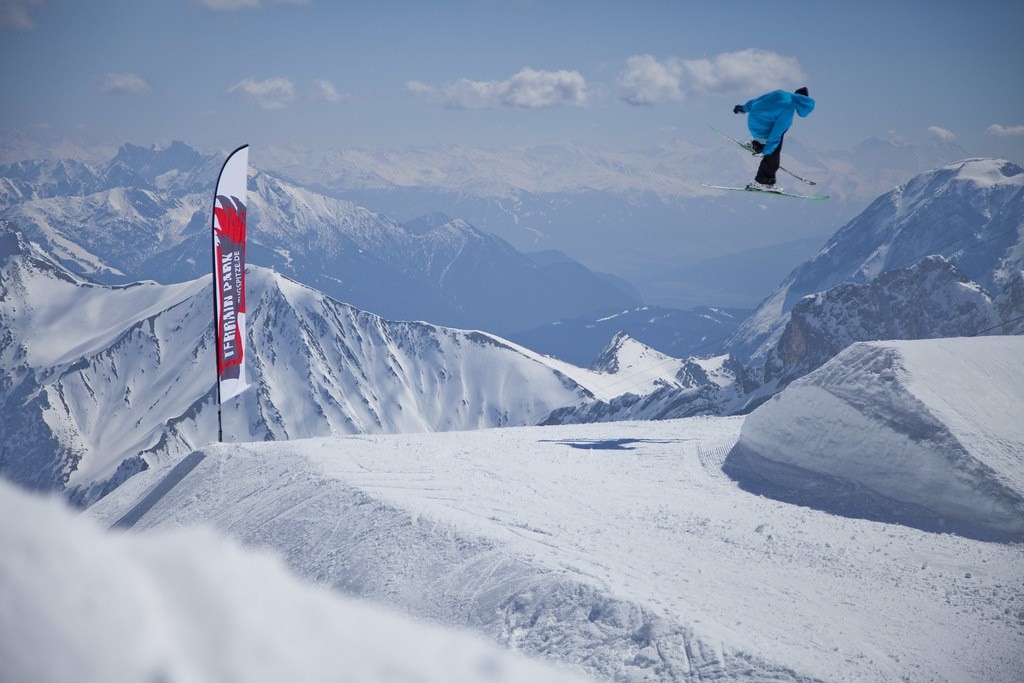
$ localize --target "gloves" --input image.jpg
[734,103,744,114]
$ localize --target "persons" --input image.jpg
[734,87,815,190]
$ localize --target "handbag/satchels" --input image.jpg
[752,140,764,153]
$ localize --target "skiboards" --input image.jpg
[698,122,832,201]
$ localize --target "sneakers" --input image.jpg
[753,180,777,190]
[752,151,765,157]
